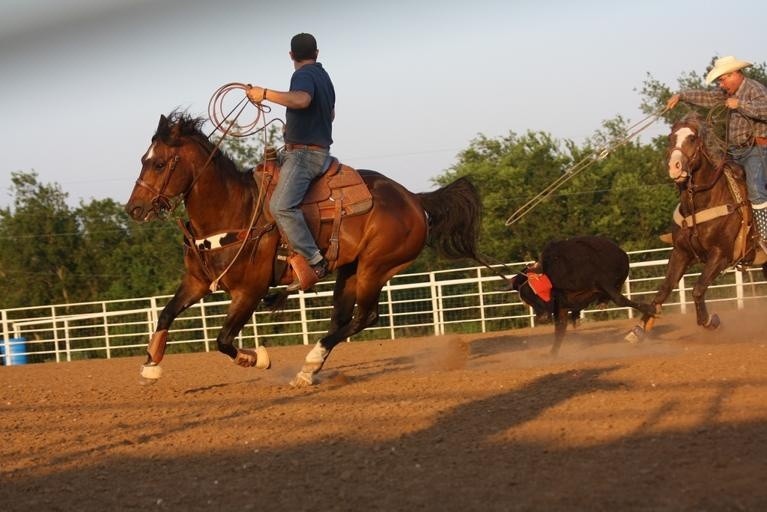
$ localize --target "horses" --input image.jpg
[124,103,528,388]
[623,111,767,347]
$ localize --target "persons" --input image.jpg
[246,31,338,296]
[665,54,766,254]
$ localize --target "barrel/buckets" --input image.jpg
[0,337,30,366]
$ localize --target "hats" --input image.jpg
[291,33,317,57]
[706,56,753,84]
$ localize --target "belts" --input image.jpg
[285,143,321,151]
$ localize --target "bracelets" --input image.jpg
[262,88,267,99]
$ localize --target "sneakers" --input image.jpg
[286,261,328,291]
[659,232,672,243]
[752,248,767,265]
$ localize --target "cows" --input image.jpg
[492,235,664,357]
